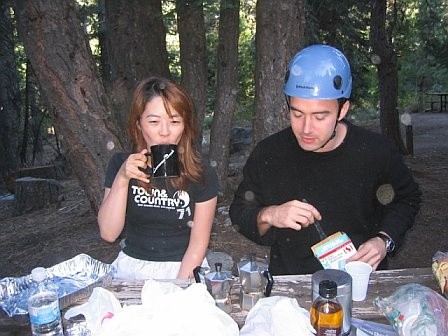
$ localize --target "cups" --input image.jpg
[139,143,181,179]
[344,261,372,302]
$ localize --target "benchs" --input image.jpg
[425,101,448,112]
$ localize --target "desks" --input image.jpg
[0,268,448,336]
[427,93,448,112]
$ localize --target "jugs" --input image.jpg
[193,262,234,312]
[237,252,274,311]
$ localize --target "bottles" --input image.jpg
[28,269,63,336]
[311,279,344,336]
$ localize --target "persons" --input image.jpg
[229,44,420,277]
[97,77,218,280]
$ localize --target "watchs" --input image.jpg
[374,232,395,253]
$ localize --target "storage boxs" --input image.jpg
[311,232,355,270]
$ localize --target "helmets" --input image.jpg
[283,45,353,99]
